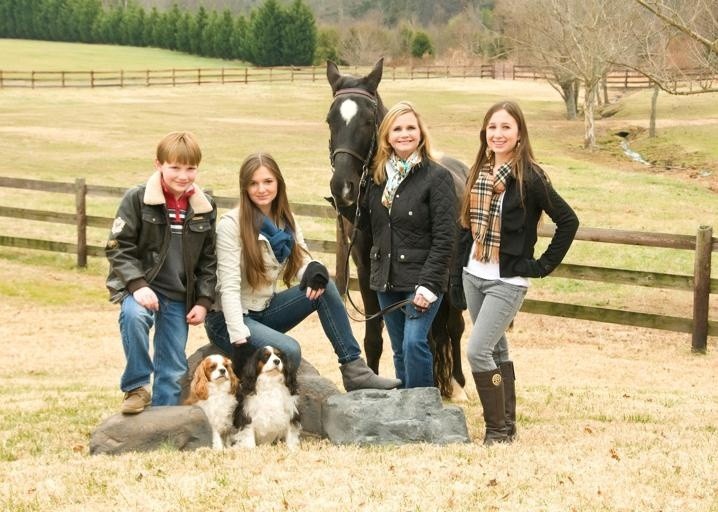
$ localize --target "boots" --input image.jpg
[472,361,516,446]
[339,358,402,392]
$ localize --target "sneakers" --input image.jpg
[121,384,152,414]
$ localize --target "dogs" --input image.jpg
[181,346,303,453]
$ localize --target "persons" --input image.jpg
[459,100,580,446]
[357,100,461,390]
[204,150,402,393]
[105,131,219,415]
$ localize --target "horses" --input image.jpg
[326,58,472,404]
